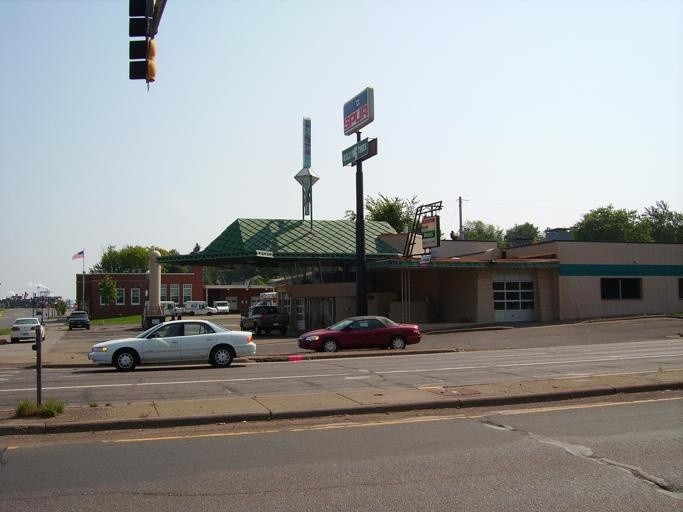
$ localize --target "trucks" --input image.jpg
[255,292,278,307]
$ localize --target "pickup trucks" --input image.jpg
[239,305,290,335]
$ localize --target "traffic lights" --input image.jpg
[128,40,155,82]
[128,1,154,38]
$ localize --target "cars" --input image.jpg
[9,317,46,343]
[66,310,90,330]
[295,314,423,355]
[87,319,256,372]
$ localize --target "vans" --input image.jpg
[142,297,230,319]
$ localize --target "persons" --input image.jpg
[170,305,176,320]
[450,230,459,241]
[177,306,181,320]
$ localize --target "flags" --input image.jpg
[71,251,82,260]
